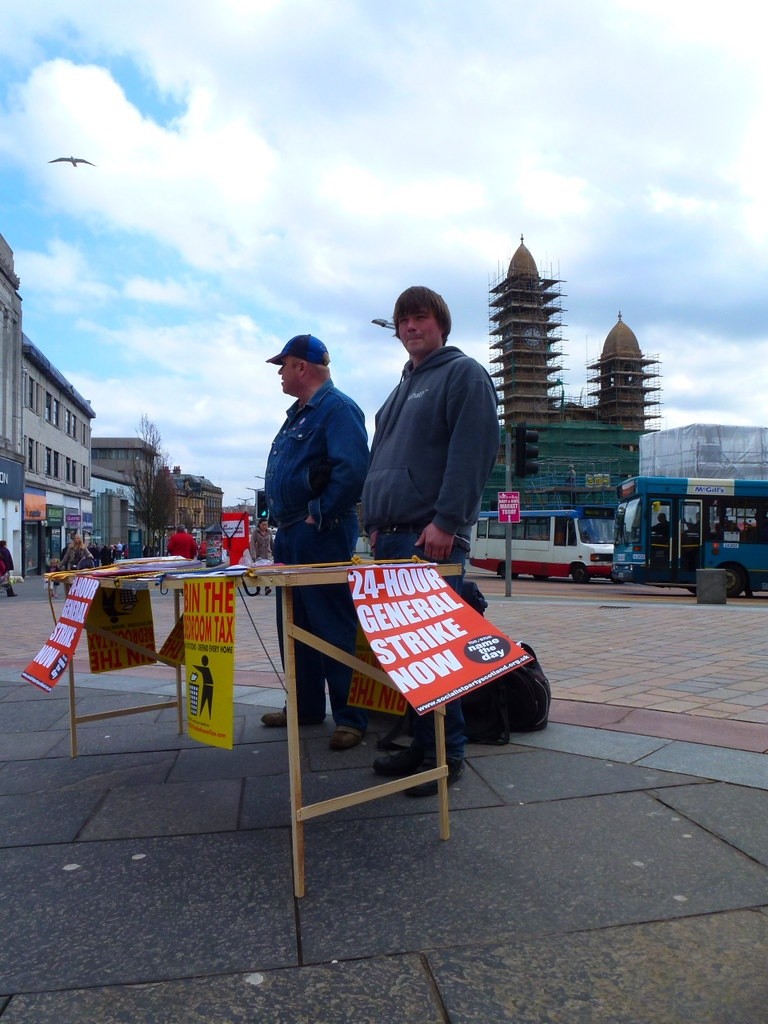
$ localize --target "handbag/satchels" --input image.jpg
[465,641,552,732]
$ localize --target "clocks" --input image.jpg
[521,325,543,349]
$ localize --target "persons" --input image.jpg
[250,519,274,595]
[262,333,370,750]
[59,535,95,589]
[0,541,19,597]
[46,559,60,599]
[167,525,198,560]
[684,512,700,532]
[60,544,72,570]
[87,540,159,568]
[652,513,670,536]
[360,286,500,797]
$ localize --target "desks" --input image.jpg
[42,561,463,899]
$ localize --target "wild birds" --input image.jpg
[48,156,96,167]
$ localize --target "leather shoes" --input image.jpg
[330,724,366,749]
[373,751,425,775]
[261,709,326,726]
[404,762,465,796]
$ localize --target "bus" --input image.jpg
[470,509,616,581]
[610,475,768,597]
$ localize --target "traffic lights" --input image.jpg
[255,490,268,519]
[513,425,540,476]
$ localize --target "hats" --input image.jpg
[266,334,331,366]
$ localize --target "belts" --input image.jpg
[379,521,426,534]
